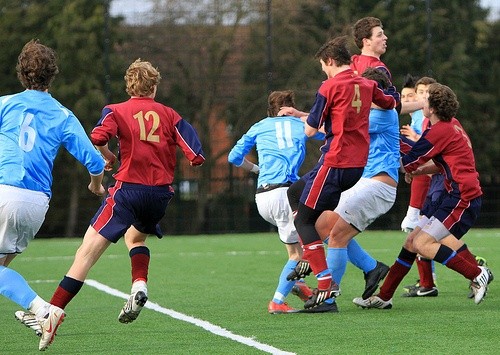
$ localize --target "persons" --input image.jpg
[400,76,487,298]
[0,38,106,349]
[401,73,438,289]
[228,90,326,314]
[276,65,401,310]
[15,58,205,338]
[352,82,494,309]
[351,16,390,79]
[287,35,400,310]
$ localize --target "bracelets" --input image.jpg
[250,164,259,173]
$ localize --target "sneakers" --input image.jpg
[34,303,66,351]
[304,281,341,309]
[287,260,312,281]
[14,311,42,337]
[268,301,293,313]
[403,280,438,297]
[468,256,488,298]
[118,290,148,324]
[305,300,337,313]
[353,295,392,309]
[469,266,494,305]
[292,282,313,302]
[362,262,390,300]
